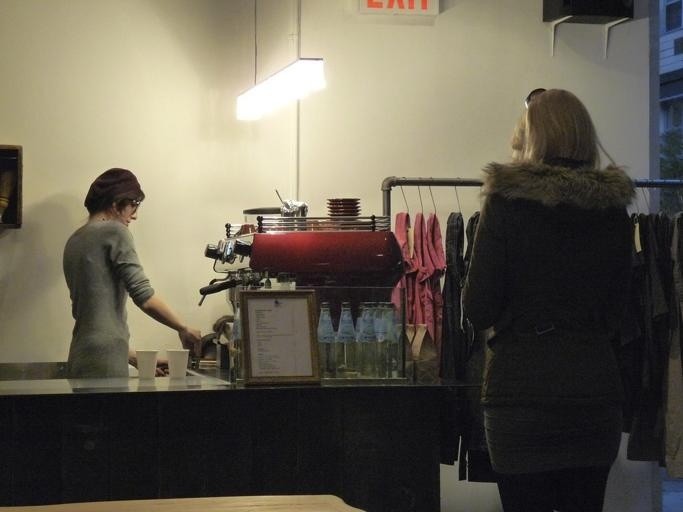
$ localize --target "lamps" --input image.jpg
[232,0,324,125]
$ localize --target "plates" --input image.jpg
[325,197,361,222]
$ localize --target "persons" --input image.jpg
[62,168,201,377]
[461,88,636,510]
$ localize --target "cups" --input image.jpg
[135,349,159,381]
[165,348,190,378]
[136,380,158,392]
[280,202,307,232]
[168,379,188,391]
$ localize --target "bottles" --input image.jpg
[316,299,398,378]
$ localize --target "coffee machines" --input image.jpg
[196,216,405,333]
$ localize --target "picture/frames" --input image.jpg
[0,144,24,229]
